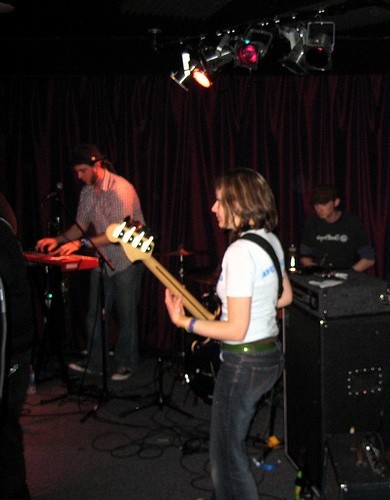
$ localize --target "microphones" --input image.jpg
[56,183,66,203]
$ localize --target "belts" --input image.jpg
[222,341,280,355]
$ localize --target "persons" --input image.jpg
[1,215,36,500]
[35,142,146,379]
[298,183,376,272]
[164,167,291,500]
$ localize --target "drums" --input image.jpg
[183,333,221,407]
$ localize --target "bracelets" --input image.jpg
[188,317,198,333]
[84,238,89,248]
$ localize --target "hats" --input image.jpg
[72,144,104,169]
[310,188,338,204]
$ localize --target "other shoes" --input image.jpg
[112,367,133,381]
[68,363,102,376]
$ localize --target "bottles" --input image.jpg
[287,243,298,272]
[27,366,36,394]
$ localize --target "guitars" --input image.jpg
[104,220,224,375]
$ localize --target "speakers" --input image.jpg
[320,432,390,500]
[281,300,390,499]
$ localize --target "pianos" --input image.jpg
[22,246,101,407]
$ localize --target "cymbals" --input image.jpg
[183,269,221,286]
[153,243,207,257]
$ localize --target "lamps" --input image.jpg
[170,6,335,92]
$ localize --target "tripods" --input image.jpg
[70,195,198,423]
